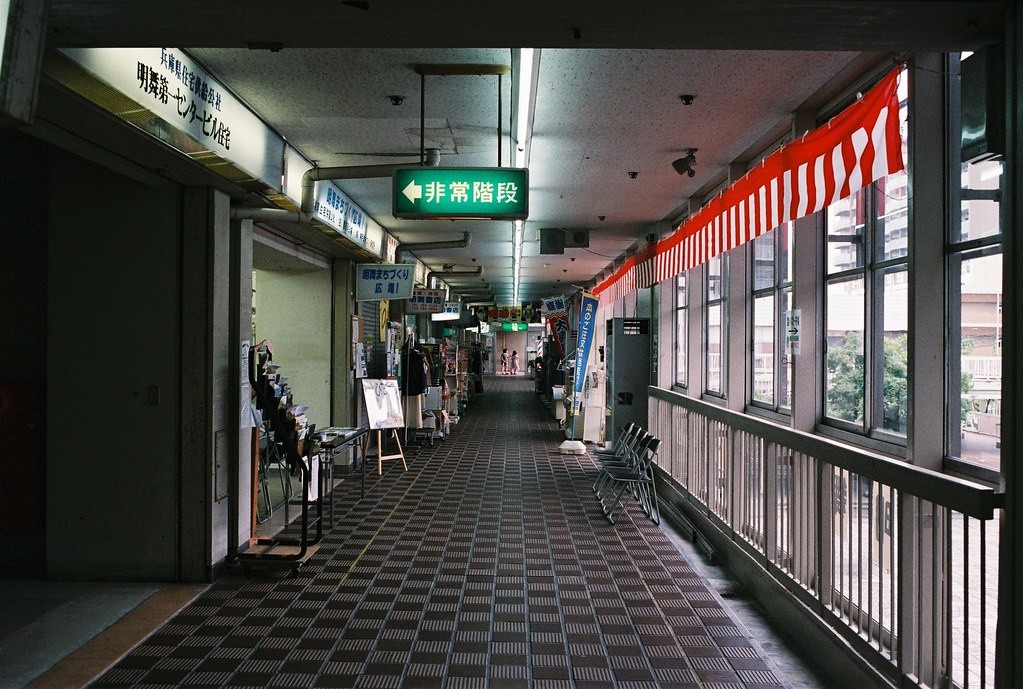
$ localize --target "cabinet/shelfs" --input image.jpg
[603,317,653,453]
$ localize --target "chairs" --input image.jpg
[591,420,663,526]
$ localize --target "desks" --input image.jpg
[284,425,369,528]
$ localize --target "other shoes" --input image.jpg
[502,372,508,375]
[510,372,517,375]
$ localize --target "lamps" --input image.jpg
[509,45,543,308]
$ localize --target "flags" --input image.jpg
[570,293,601,418]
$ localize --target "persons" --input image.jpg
[445,363,456,374]
[510,350,520,375]
[500,349,509,375]
